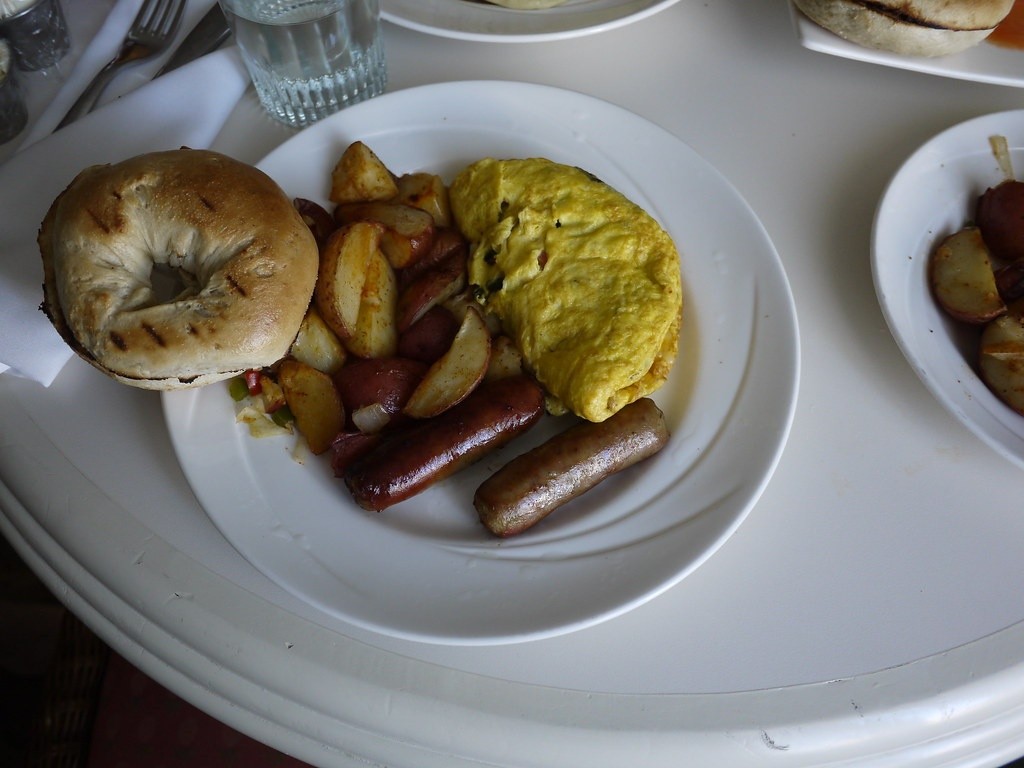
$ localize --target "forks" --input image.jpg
[51,0,188,135]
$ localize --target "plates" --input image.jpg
[871,110,1024,473]
[159,81,801,645]
[380,0,680,43]
[792,1,1024,90]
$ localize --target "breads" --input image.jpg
[36,147,320,390]
[794,0,1015,57]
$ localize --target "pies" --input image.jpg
[449,157,683,423]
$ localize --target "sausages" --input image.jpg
[350,382,668,537]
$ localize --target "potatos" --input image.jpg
[278,141,522,456]
[932,179,1024,413]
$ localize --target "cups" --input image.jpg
[0,0,69,72]
[219,0,388,129]
[0,43,28,145]
